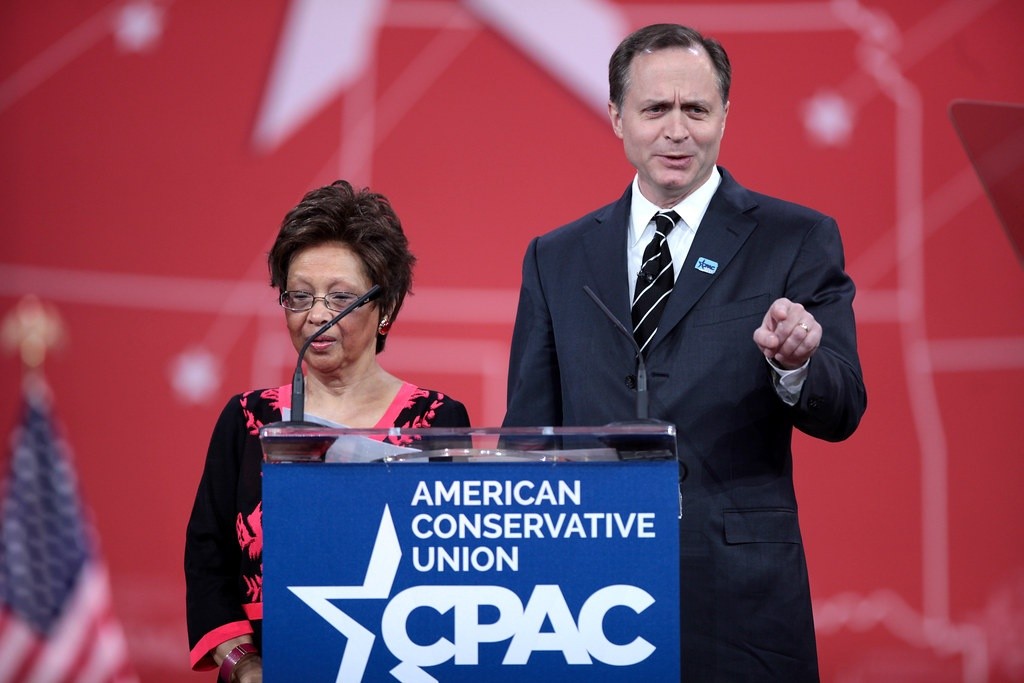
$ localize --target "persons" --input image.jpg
[498,22,868,683]
[184,179,473,683]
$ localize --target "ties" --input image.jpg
[632,211,682,370]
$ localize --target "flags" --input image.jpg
[0,357,139,683]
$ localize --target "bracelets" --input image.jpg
[216,643,258,683]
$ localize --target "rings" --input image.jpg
[797,323,809,333]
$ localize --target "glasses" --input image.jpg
[279,290,379,313]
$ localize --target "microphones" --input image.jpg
[584,286,664,427]
[645,261,659,283]
[259,284,381,432]
